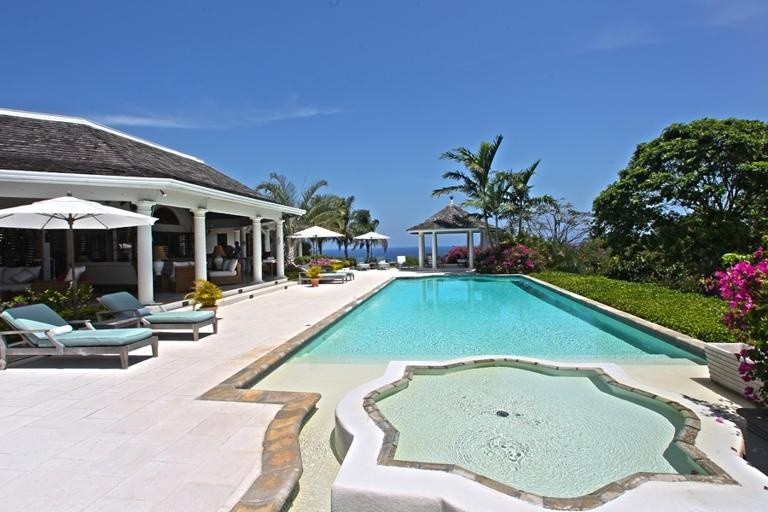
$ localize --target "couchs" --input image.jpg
[170,258,245,288]
[0,263,94,292]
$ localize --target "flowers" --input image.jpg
[709,244,768,416]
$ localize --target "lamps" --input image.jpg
[152,246,169,276]
[211,245,224,269]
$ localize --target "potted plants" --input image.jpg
[183,279,223,321]
[306,264,321,287]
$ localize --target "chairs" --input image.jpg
[1,300,164,370]
[93,292,223,341]
[297,264,356,285]
[355,255,411,271]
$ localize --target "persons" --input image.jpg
[233,241,241,262]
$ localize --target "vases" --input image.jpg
[736,402,767,443]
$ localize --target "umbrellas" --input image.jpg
[353,231,392,262]
[0,191,159,318]
[286,225,345,261]
